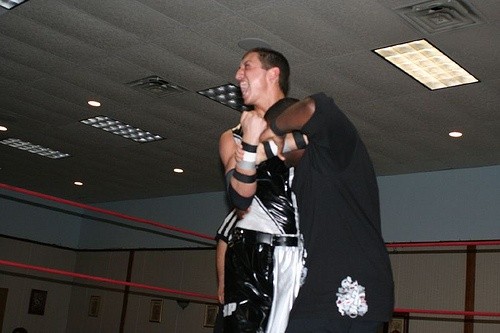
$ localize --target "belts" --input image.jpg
[235,226,298,246]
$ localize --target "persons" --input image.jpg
[259,92,395,333]
[213,203,250,333]
[219,46,310,333]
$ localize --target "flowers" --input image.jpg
[336,276,368,316]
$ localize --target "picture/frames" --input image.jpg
[148,298,164,323]
[28,289,48,315]
[88,296,101,317]
[203,304,219,328]
[385,313,409,333]
